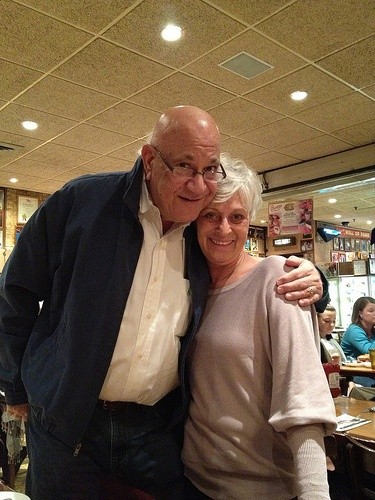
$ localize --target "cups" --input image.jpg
[369,347,375,371]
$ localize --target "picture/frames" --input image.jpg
[330,237,367,262]
[244,237,259,257]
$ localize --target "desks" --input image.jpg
[333,361,375,440]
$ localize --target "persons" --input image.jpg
[0,105,330,500]
[317,304,375,402]
[341,297,375,387]
[181,154,337,500]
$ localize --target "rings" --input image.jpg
[310,288,314,295]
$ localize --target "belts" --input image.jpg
[96,399,150,414]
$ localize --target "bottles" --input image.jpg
[321,363,341,405]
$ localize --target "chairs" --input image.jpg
[333,433,375,500]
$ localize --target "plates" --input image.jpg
[0,491,31,500]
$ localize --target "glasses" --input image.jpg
[153,145,227,183]
